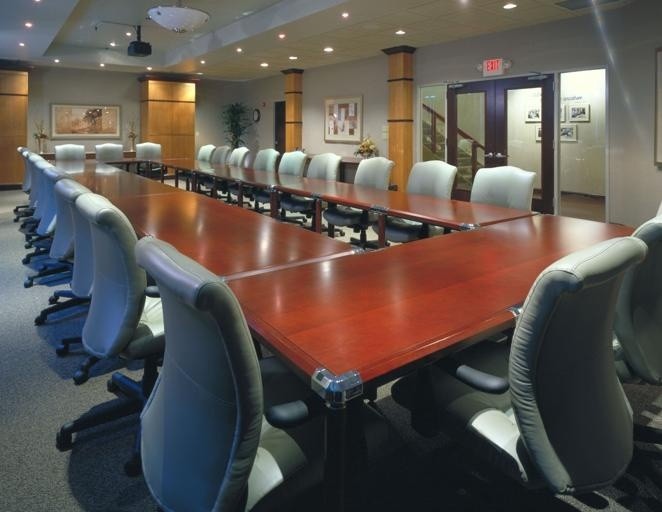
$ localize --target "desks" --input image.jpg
[220,214,636,512]
[274,177,540,231]
[193,164,312,222]
[109,192,364,290]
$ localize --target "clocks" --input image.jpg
[253,109,260,121]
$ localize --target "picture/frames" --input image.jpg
[560,104,566,121]
[559,123,577,142]
[49,103,122,140]
[653,48,662,165]
[535,125,541,142]
[568,103,590,122]
[524,107,541,123]
[323,93,364,144]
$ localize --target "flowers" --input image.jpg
[353,135,378,158]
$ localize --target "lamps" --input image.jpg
[147,1,209,33]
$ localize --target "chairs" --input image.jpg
[505,214,661,447]
[371,159,460,245]
[324,155,398,252]
[280,150,346,238]
[467,163,538,213]
[132,236,366,512]
[14,141,263,448]
[387,232,650,511]
[249,147,280,213]
[275,150,309,225]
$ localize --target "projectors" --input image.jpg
[128,41,152,57]
